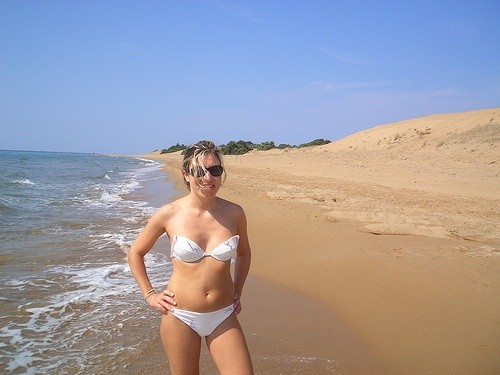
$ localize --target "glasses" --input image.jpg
[188,165,224,177]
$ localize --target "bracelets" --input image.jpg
[144,288,157,303]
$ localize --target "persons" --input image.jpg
[129,140,256,375]
